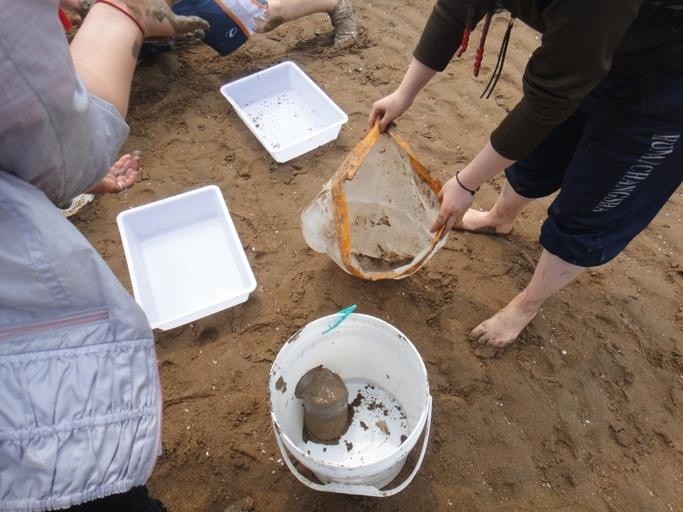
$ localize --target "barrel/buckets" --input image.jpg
[266,310,435,498]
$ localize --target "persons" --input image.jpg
[168,0,360,51]
[365,0,683,349]
[2,1,168,511]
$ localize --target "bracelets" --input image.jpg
[454,170,480,196]
[94,0,151,38]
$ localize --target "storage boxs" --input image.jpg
[219,60,349,164]
[116,184,258,333]
